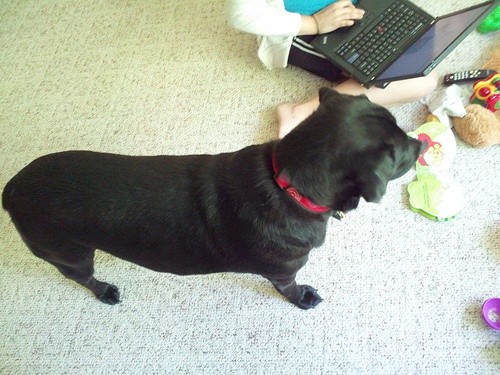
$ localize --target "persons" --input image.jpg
[227,0,438,140]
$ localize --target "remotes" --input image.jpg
[445,70,496,84]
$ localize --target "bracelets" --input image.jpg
[312,14,320,35]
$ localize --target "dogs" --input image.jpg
[2,86,424,311]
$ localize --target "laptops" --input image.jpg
[311,0,500,89]
